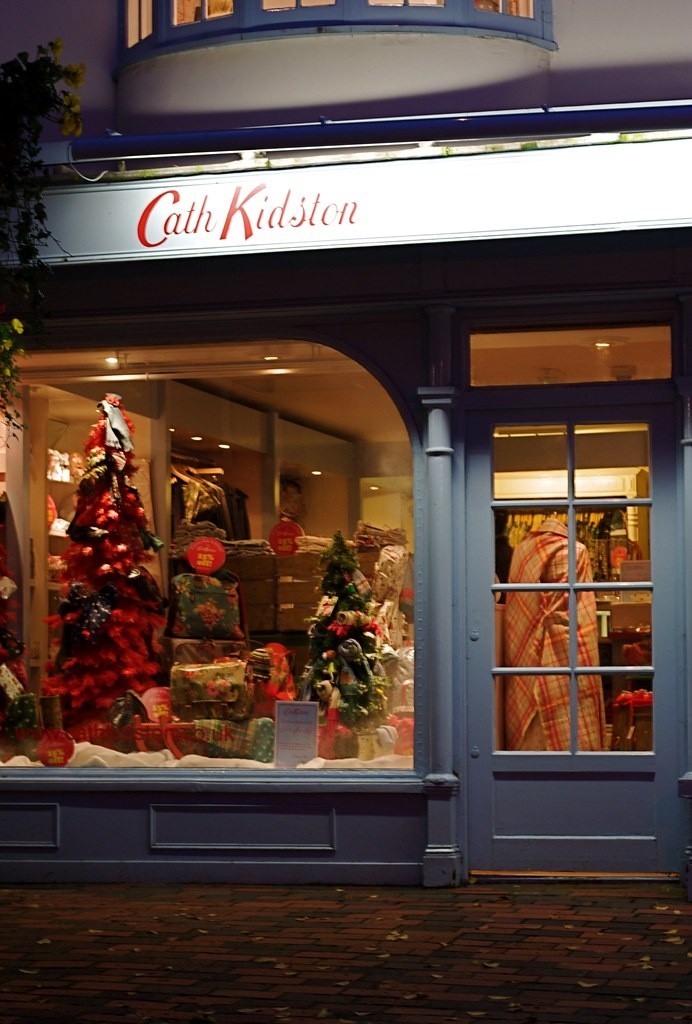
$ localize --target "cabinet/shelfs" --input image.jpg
[610,598,654,753]
[29,475,99,668]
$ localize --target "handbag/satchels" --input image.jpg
[169,660,252,722]
[162,571,245,639]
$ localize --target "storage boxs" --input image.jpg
[108,545,415,761]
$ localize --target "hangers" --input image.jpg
[506,513,605,530]
[170,463,226,494]
